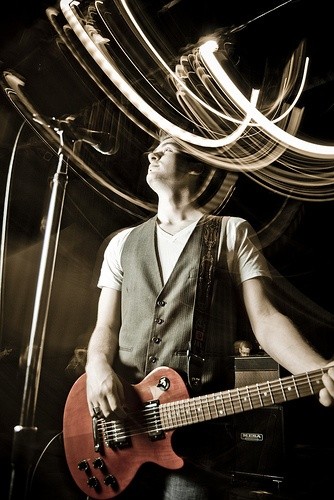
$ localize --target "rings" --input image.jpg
[93,406,102,415]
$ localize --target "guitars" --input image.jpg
[61,360,334,500]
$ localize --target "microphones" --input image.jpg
[32,114,120,155]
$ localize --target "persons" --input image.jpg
[238,341,252,357]
[85,130,334,500]
